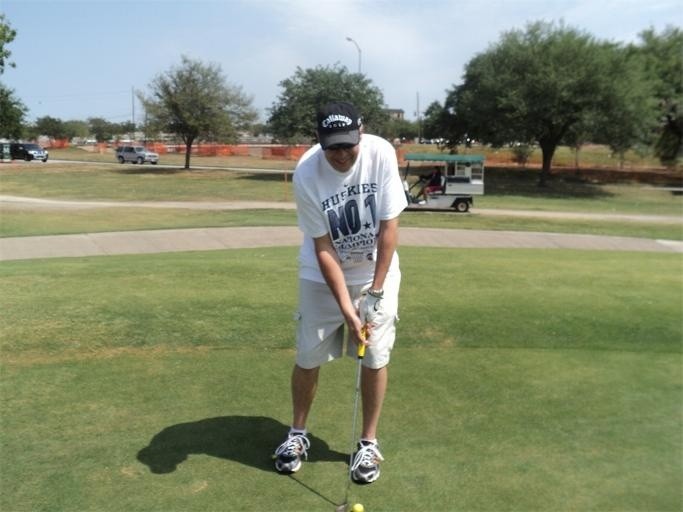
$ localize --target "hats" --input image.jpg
[318,102,361,150]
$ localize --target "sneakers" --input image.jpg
[352,441,380,483]
[275,432,306,473]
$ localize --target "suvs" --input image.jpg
[115,144,159,164]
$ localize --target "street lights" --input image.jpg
[346,37,361,73]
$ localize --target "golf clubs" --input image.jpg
[336,327,365,512]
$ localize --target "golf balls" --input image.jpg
[353,504,364,512]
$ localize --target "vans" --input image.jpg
[10,143,48,161]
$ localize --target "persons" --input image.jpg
[415,165,444,205]
[275,101,408,484]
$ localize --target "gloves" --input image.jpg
[359,290,383,325]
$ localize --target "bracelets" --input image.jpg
[369,286,386,297]
